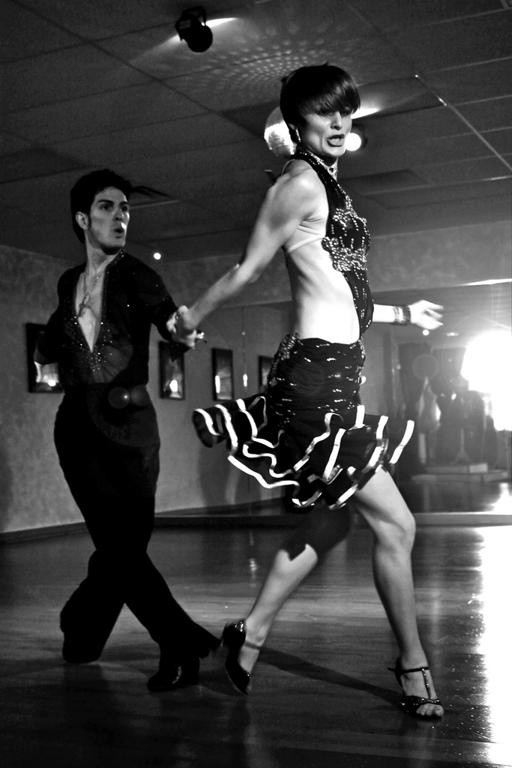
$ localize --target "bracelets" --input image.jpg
[392,304,409,327]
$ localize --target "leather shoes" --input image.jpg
[164,657,200,691]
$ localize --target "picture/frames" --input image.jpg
[212,349,234,401]
[159,341,185,400]
[259,357,274,393]
[27,323,63,394]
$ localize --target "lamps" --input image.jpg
[175,7,212,52]
[347,125,365,152]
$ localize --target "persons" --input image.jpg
[172,62,449,723]
[38,168,224,695]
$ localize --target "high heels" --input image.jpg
[215,618,255,695]
[388,654,445,721]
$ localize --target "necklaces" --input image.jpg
[77,266,103,318]
